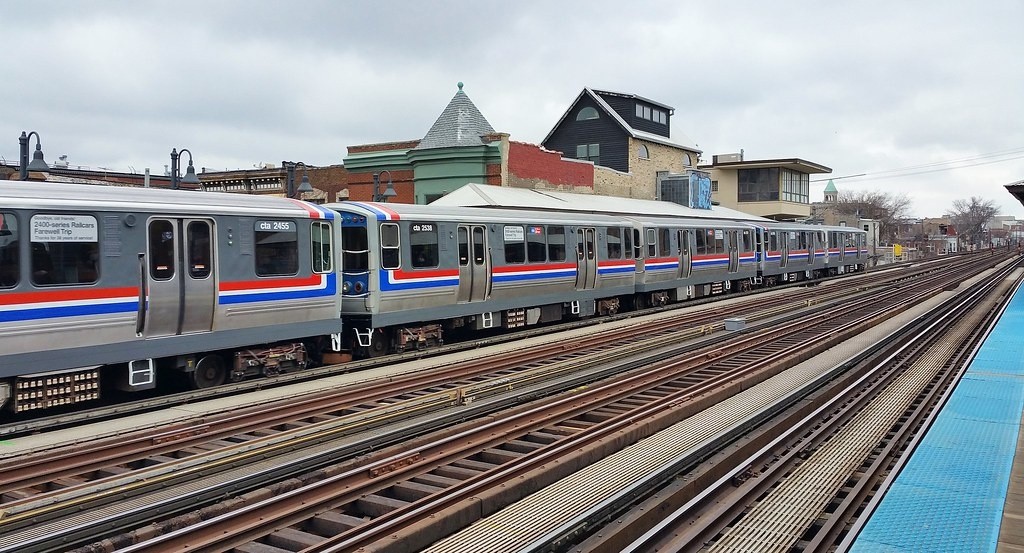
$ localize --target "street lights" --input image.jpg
[19,129,51,182]
[167,147,203,192]
[279,161,315,201]
[372,170,398,203]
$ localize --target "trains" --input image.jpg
[0,179,870,431]
